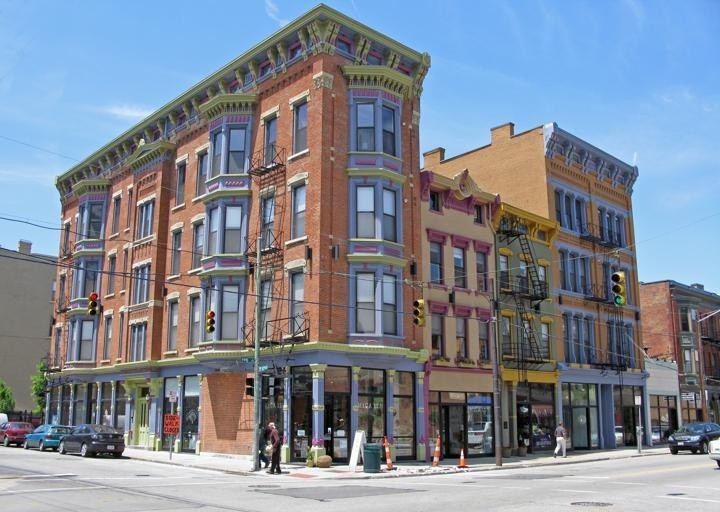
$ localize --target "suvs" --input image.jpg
[668,422,720,468]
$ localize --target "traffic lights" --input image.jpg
[611,271,628,307]
[205,310,215,333]
[86,292,98,318]
[413,298,427,328]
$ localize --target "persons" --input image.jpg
[536,423,545,435]
[258,423,270,468]
[265,421,282,474]
[553,421,567,457]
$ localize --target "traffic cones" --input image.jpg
[384,434,468,471]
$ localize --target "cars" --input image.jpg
[615,426,666,445]
[468,421,492,448]
[0,422,125,457]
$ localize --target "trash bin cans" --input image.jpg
[363,443,381,473]
